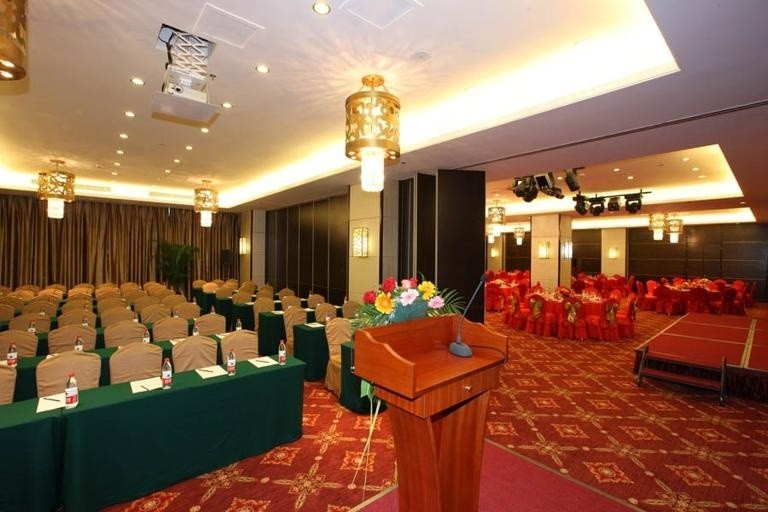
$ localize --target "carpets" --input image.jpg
[347,435,646,512]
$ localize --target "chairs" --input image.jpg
[484,268,637,341]
[633,276,756,319]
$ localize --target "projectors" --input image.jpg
[163,81,208,104]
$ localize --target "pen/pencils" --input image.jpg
[199,369,213,372]
[43,398,60,401]
[141,385,149,391]
[256,360,269,362]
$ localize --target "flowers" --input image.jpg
[349,275,463,338]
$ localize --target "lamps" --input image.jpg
[648,211,667,241]
[351,227,368,259]
[193,177,219,227]
[563,166,584,191]
[0,0,28,81]
[344,73,401,194]
[485,198,574,260]
[35,158,75,220]
[607,247,617,260]
[510,175,537,203]
[665,214,684,244]
[571,188,651,217]
[238,237,247,256]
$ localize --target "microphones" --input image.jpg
[449,272,489,357]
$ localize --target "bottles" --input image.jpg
[142,328,150,344]
[278,340,286,365]
[235,318,242,331]
[64,372,79,410]
[82,318,88,328]
[7,340,18,368]
[28,318,36,333]
[227,349,236,376]
[210,304,215,314]
[162,358,172,390]
[193,296,196,304]
[131,310,138,322]
[192,322,199,336]
[173,309,179,318]
[74,334,83,352]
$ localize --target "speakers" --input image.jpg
[534,174,553,191]
